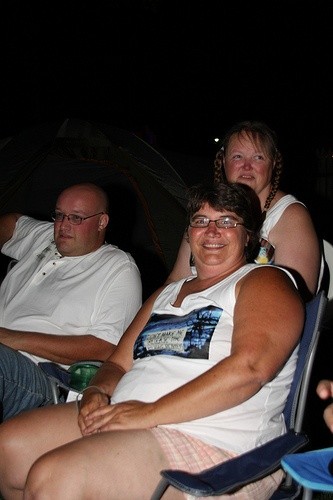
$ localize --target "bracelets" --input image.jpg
[75,384,104,413]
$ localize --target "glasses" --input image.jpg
[189,214,246,228]
[51,211,104,225]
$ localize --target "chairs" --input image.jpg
[38,290,329,499]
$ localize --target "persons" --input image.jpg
[316,380,333,434]
[0,185,141,420]
[165,116,319,297]
[0,183,305,500]
